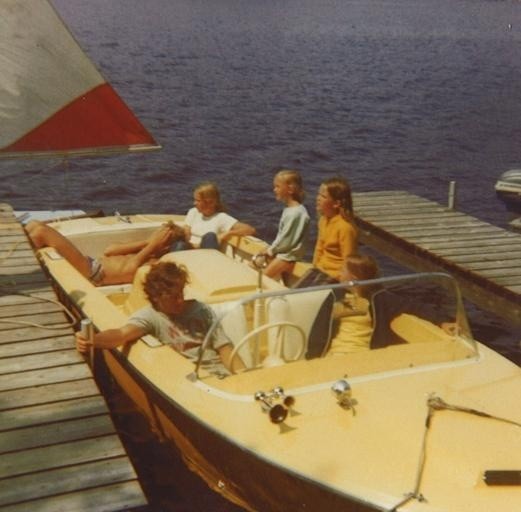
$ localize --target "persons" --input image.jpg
[290,180,358,289]
[257,172,311,277]
[74,262,246,381]
[22,219,183,287]
[318,253,385,358]
[171,184,256,253]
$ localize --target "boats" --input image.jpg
[34,214,521,512]
